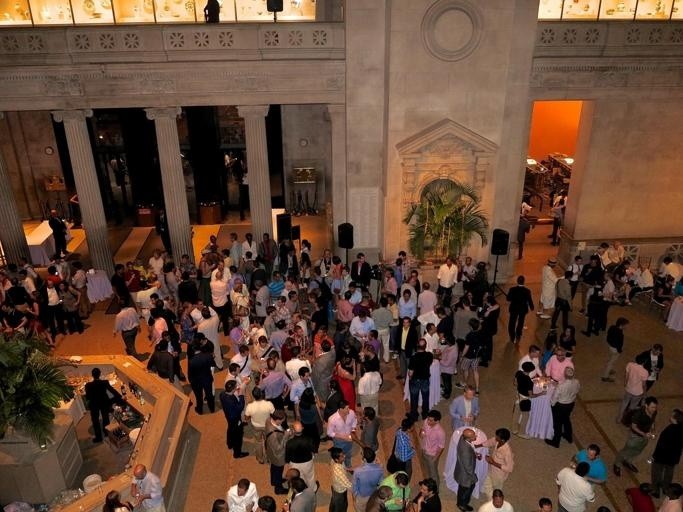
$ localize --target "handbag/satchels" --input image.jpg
[387,455,405,474]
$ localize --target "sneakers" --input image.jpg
[455,382,465,388]
[457,502,474,510]
[510,336,521,347]
[92,437,104,444]
[49,329,85,349]
[537,311,552,321]
[607,297,633,307]
[193,394,216,415]
[602,369,617,383]
[549,325,608,336]
[475,390,481,397]
[227,442,290,494]
[512,427,573,448]
[403,384,449,420]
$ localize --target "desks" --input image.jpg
[25,220,72,267]
[519,148,574,225]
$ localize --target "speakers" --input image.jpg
[491,229,509,255]
[338,223,353,248]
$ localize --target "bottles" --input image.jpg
[120,383,125,395]
[127,380,145,405]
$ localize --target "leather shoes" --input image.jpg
[613,459,623,478]
[625,461,638,474]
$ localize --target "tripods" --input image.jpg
[487,255,508,299]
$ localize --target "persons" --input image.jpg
[110,156,128,186]
[0,231,683,512]
[224,155,244,181]
[154,208,171,256]
[48,210,70,259]
[517,188,567,259]
